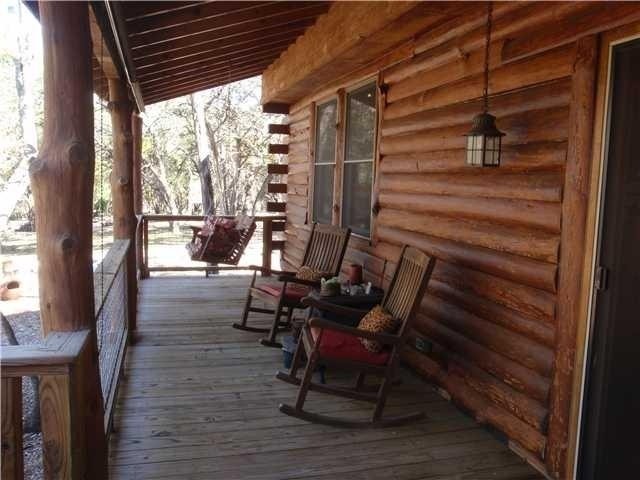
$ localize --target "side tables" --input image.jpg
[308,286,383,321]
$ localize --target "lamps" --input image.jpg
[461,0,507,168]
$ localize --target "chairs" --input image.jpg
[232,222,350,347]
[278,244,437,429]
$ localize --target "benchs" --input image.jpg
[185,214,257,265]
